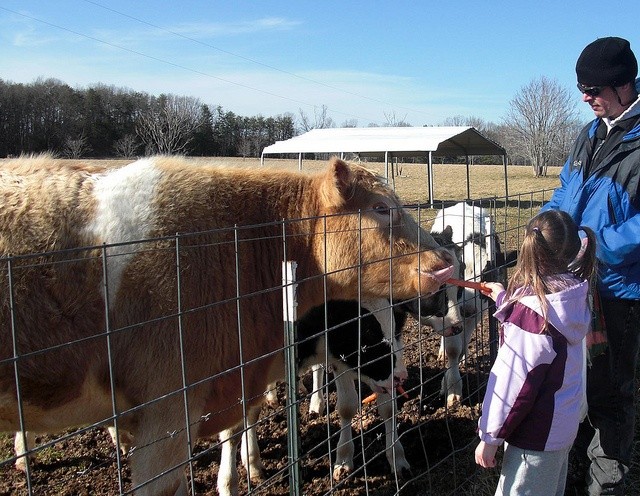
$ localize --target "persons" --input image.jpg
[540,36,640,496]
[474,209,596,496]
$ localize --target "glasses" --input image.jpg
[576,83,602,96]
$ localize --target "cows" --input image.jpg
[425,202,506,416]
[15,295,409,496]
[0,150,458,496]
[265,222,467,480]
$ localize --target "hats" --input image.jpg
[575,37,638,86]
